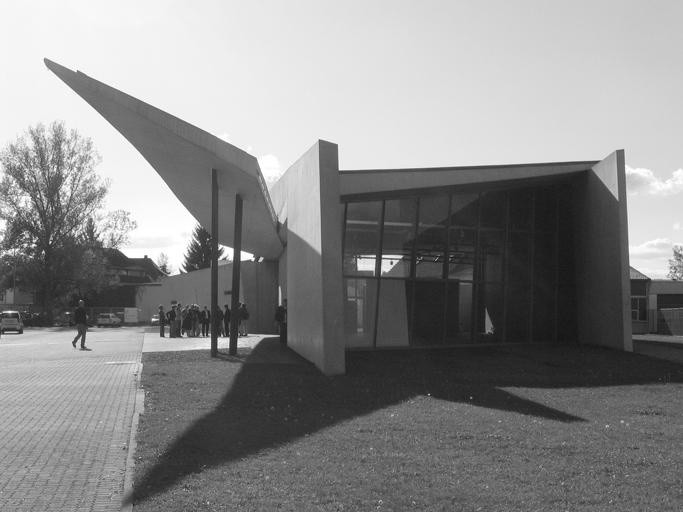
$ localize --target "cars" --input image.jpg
[150,315,159,325]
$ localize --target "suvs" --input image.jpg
[96,312,122,327]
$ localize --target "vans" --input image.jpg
[0,310,23,334]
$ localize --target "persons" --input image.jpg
[275,300,287,345]
[159,301,250,338]
[72,300,89,348]
